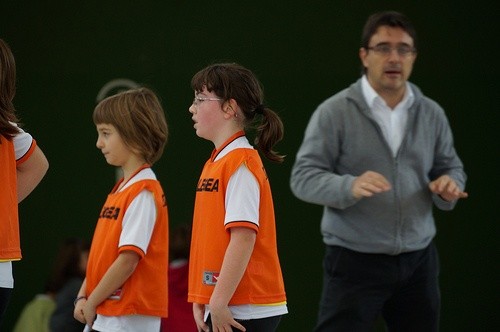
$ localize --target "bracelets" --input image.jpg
[74,296,88,304]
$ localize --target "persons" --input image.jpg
[1,40,50,331]
[289,11,467,332]
[73,88,170,331]
[186,61,289,331]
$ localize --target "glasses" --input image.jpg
[365,44,416,56]
[194,97,225,104]
